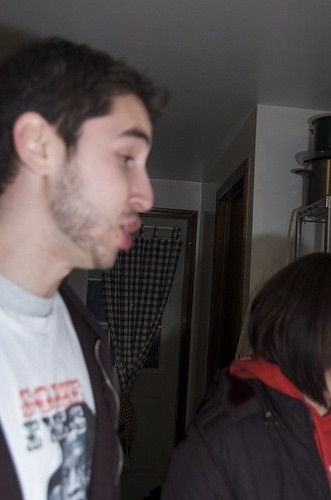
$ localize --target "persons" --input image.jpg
[161,252,331,500]
[0,33,155,500]
